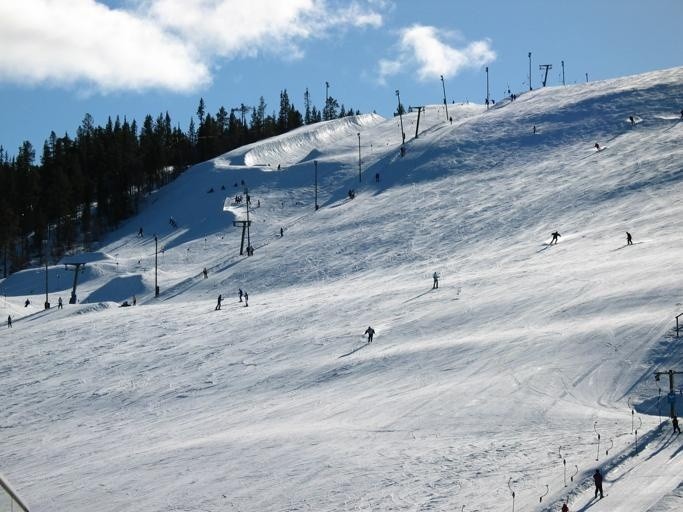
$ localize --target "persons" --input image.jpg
[624,231,633,246]
[671,416,681,434]
[594,141,600,152]
[591,468,604,500]
[364,326,376,343]
[243,290,248,307]
[212,295,224,311]
[132,295,136,305]
[201,267,207,279]
[249,244,254,255]
[629,114,635,127]
[6,315,11,328]
[431,270,440,288]
[551,230,561,245]
[237,288,243,303]
[57,296,62,310]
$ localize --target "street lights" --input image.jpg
[527,50,532,92]
[42,257,50,309]
[484,65,490,110]
[438,73,448,120]
[240,185,254,257]
[559,59,565,85]
[393,88,405,144]
[582,72,589,82]
[153,235,159,297]
[313,160,319,211]
[355,131,362,184]
[323,79,330,99]
